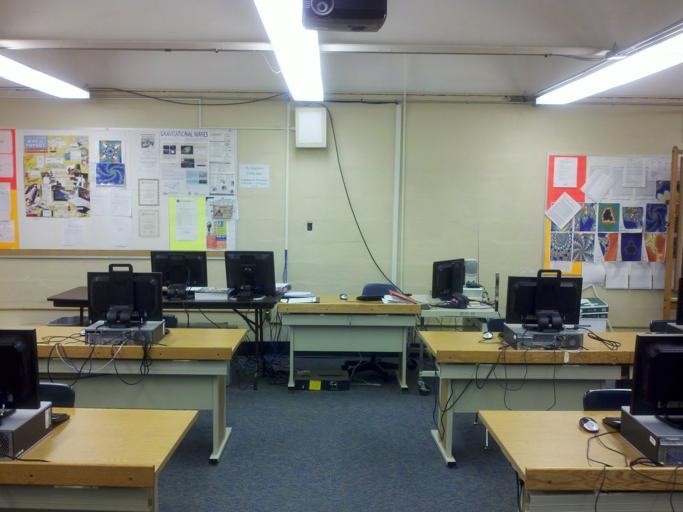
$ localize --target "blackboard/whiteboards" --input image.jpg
[0,127,237,253]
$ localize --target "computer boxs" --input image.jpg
[665,323,683,334]
[1,400,54,461]
[502,322,583,350]
[84,320,166,345]
[621,406,683,466]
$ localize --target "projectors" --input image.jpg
[302,1,387,31]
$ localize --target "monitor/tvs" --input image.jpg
[224,251,275,301]
[676,278,683,325]
[505,270,583,332]
[432,258,469,308]
[1,328,40,424]
[150,251,207,299]
[629,334,683,430]
[87,264,162,328]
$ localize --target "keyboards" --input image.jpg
[52,412,70,425]
[603,416,620,429]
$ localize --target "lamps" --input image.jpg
[531,17,681,104]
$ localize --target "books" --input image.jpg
[381,289,417,305]
[284,291,320,303]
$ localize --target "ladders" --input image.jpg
[664,146,683,321]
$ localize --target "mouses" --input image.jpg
[340,293,348,299]
[80,328,86,335]
[483,331,493,339]
[579,417,598,433]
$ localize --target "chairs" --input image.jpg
[38,381,77,409]
[583,387,629,411]
[341,283,411,383]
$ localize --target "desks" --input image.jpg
[0,407,199,510]
[410,326,656,468]
[46,283,284,390]
[276,293,421,392]
[1,325,248,465]
[476,405,682,512]
[414,297,500,396]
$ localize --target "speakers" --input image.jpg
[464,259,477,285]
[295,106,326,148]
